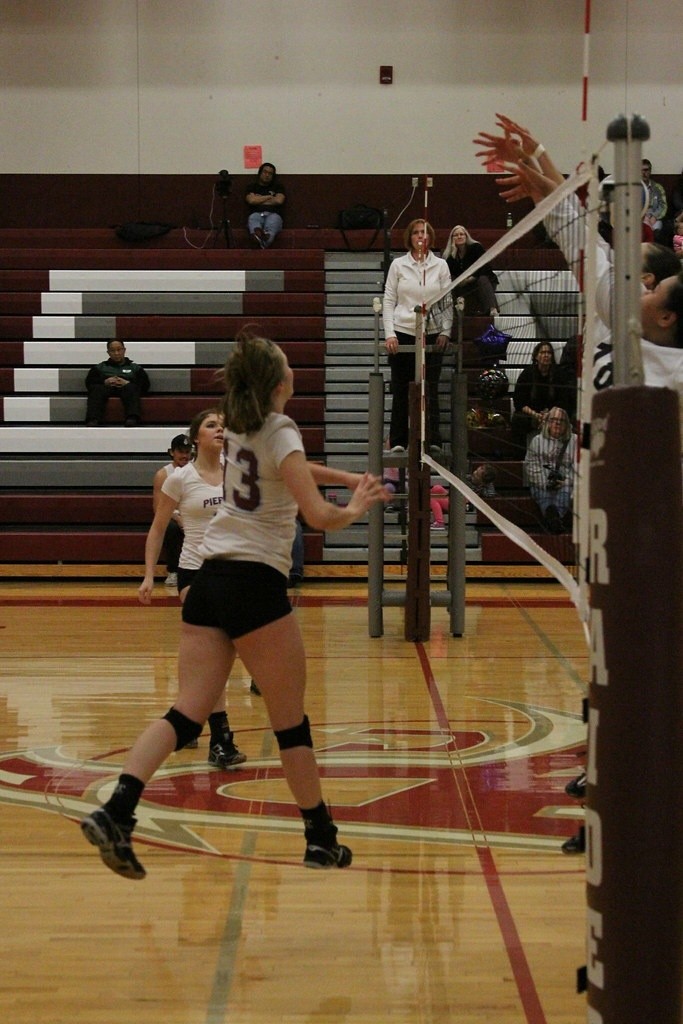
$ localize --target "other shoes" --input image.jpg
[286,574,302,587]
[428,440,442,452]
[87,416,100,427]
[562,825,585,855]
[125,414,141,426]
[390,441,408,452]
[384,503,394,512]
[545,505,572,534]
[565,773,587,797]
[251,234,267,249]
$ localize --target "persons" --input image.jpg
[243,163,288,250]
[383,220,454,453]
[472,112,683,854]
[525,407,578,534]
[559,334,578,378]
[511,343,577,460]
[430,464,495,531]
[250,679,262,696]
[286,519,303,588]
[383,432,409,513]
[81,336,393,880]
[153,434,194,586]
[442,225,500,316]
[138,408,247,766]
[85,339,150,427]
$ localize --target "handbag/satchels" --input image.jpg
[338,202,384,229]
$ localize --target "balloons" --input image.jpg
[475,325,510,363]
[476,369,509,401]
[467,408,509,434]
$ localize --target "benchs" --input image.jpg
[0,225,585,567]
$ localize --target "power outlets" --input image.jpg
[426,177,433,187]
[412,177,419,188]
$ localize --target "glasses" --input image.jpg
[108,348,125,354]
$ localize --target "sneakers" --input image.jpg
[184,735,198,748]
[250,682,262,695]
[304,842,354,869]
[164,572,178,587]
[430,521,445,531]
[81,808,146,880]
[208,732,247,766]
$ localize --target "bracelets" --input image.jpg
[531,143,545,175]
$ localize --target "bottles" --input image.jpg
[329,495,336,505]
[506,213,513,230]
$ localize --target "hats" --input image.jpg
[171,434,192,449]
